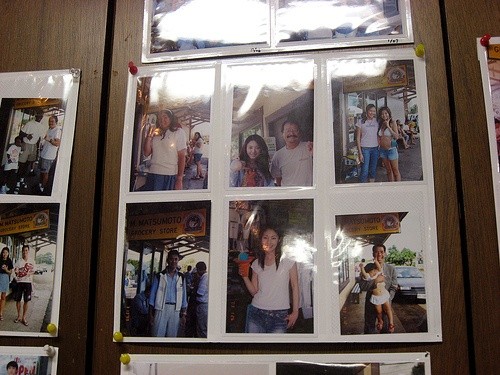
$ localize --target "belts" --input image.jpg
[163,302,176,305]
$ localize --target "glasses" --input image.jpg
[284,128,298,133]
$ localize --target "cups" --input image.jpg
[238,261,249,276]
[148,124,155,136]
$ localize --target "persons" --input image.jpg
[355,104,418,182]
[230,135,271,187]
[4,136,24,195]
[191,132,204,179]
[7,361,18,375]
[359,256,394,332]
[240,224,299,333]
[359,244,398,334]
[269,119,313,186]
[0,247,14,321]
[37,116,62,192]
[144,107,185,191]
[12,245,32,326]
[146,250,208,338]
[16,110,44,190]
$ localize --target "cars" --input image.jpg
[34,267,43,274]
[393,266,427,304]
